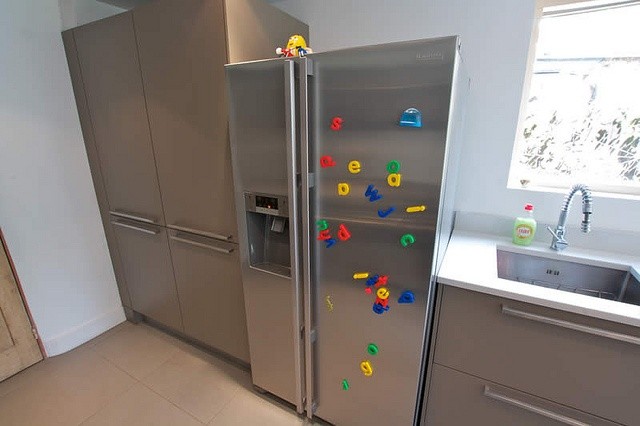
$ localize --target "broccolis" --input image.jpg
[224,34,471,426]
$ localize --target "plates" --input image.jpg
[60,0,309,366]
[423,285,640,426]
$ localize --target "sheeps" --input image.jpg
[545,183,593,251]
